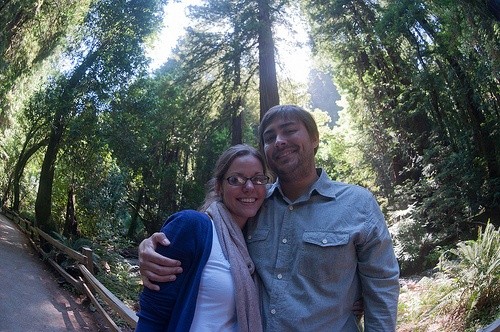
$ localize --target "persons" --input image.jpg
[138,104,400,331]
[133,144,269,331]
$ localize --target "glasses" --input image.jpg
[223,175,269,186]
